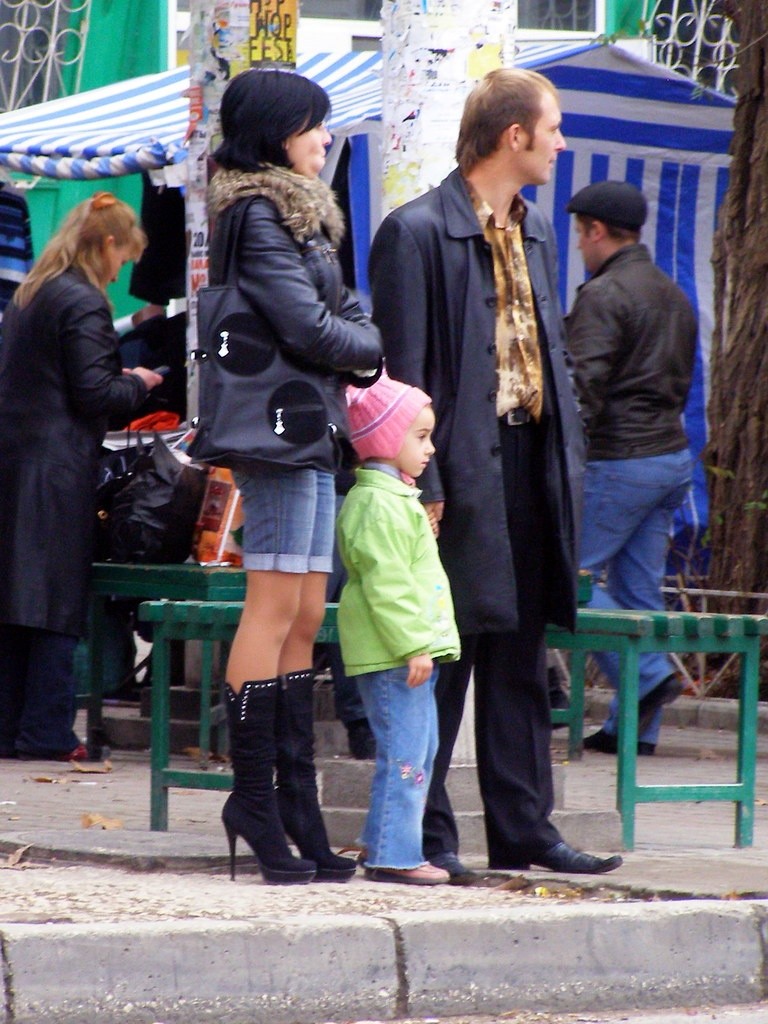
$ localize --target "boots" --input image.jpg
[221,677,317,884]
[272,668,358,882]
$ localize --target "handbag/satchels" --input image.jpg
[189,195,344,475]
[94,424,208,562]
[191,465,246,567]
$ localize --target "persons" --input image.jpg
[366,66,623,883]
[0,190,165,764]
[201,72,380,884]
[563,180,698,754]
[336,376,462,883]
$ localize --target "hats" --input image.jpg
[565,180,647,232]
[344,375,433,460]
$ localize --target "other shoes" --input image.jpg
[348,723,378,758]
[358,848,372,867]
[638,675,684,717]
[17,743,111,762]
[584,731,654,756]
[368,862,449,884]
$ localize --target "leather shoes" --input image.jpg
[489,842,623,872]
[427,851,476,885]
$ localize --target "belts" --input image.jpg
[497,407,533,426]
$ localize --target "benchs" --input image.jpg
[69,560,244,761]
[137,595,768,854]
